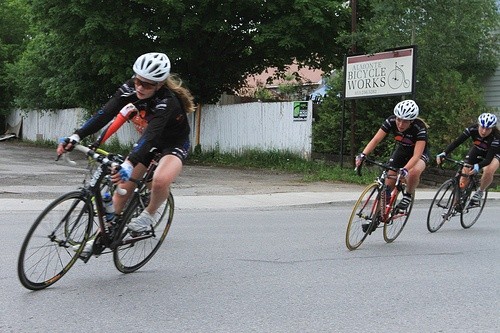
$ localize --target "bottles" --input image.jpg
[102,193,117,224]
[459,183,467,194]
[385,184,391,205]
[90,197,98,216]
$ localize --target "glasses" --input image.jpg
[131,74,161,90]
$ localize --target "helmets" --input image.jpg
[132,52,171,82]
[478,113,497,129]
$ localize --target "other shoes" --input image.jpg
[396,194,412,210]
[362,220,377,231]
[472,189,484,201]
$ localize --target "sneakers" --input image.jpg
[128,208,157,232]
[394,100,419,120]
[72,240,102,258]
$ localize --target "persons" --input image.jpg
[57,52,196,252]
[436,113,500,218]
[355,100,429,231]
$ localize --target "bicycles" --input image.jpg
[345,153,416,251]
[425,154,490,234]
[16,138,178,291]
